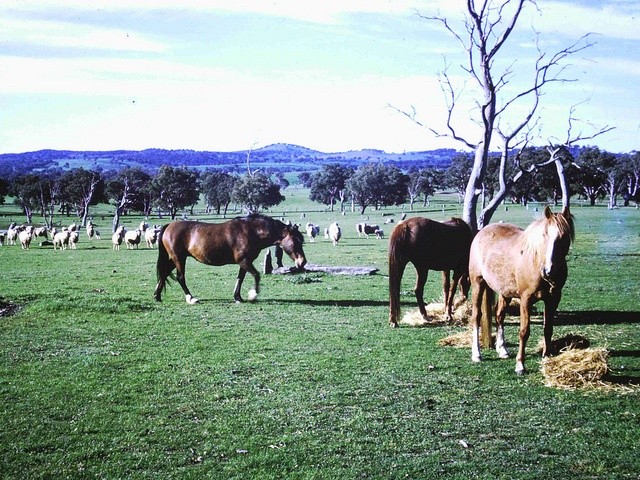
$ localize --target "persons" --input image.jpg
[275,242,283,267]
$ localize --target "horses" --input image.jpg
[467,205,577,377]
[152,212,307,304]
[387,217,472,328]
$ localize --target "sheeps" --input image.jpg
[50,227,60,239]
[68,223,77,232]
[139,221,149,232]
[53,231,70,250]
[356,224,363,238]
[6,222,48,249]
[116,225,126,239]
[363,222,379,239]
[375,229,384,239]
[145,227,157,248]
[123,229,141,250]
[68,232,79,249]
[112,233,122,251]
[86,225,94,239]
[329,222,341,247]
[0,231,8,246]
[307,226,317,242]
